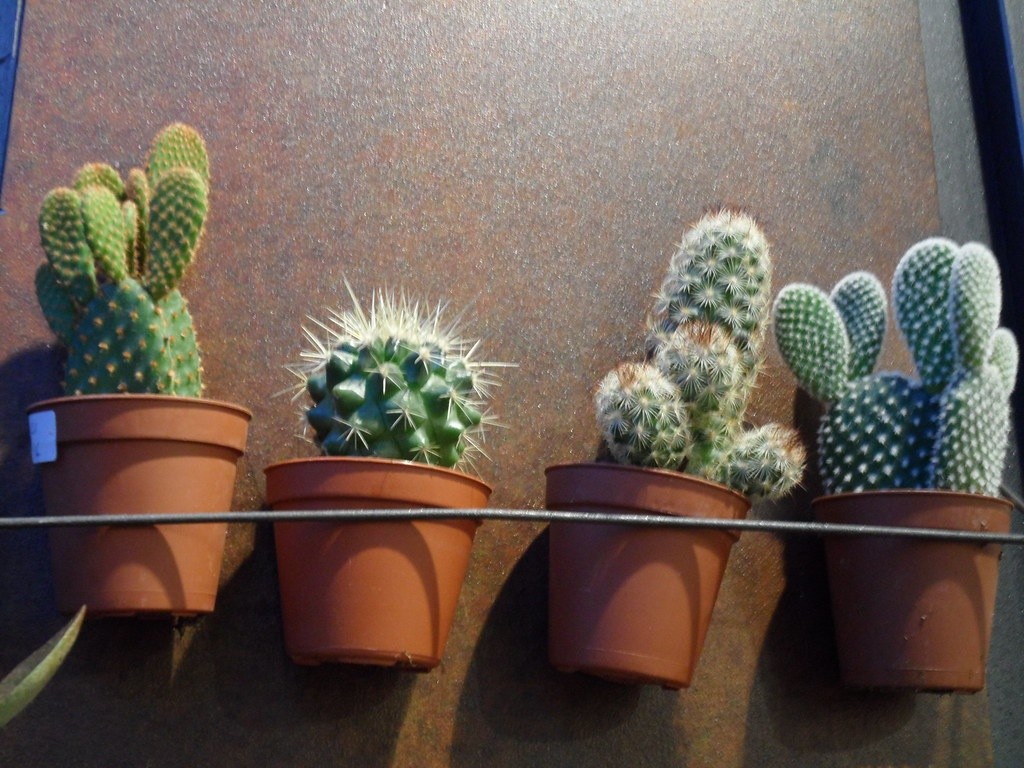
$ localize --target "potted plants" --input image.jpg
[545,212,808,690]
[25,122,251,618]
[262,271,521,672]
[773,236,1018,696]
